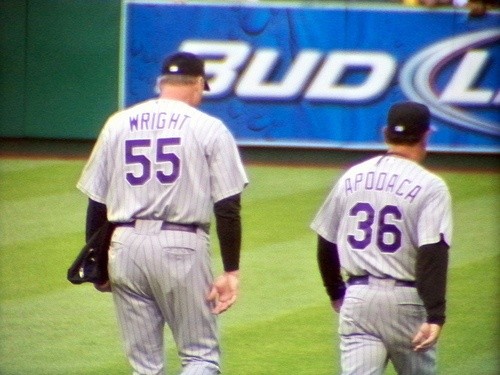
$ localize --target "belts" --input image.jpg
[348,275,418,288]
[114,220,197,234]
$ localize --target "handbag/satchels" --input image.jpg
[67,222,112,285]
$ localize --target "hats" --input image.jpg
[161,51,211,91]
[388,103,431,134]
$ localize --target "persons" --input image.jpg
[310,101,453,375]
[78,52,249,375]
